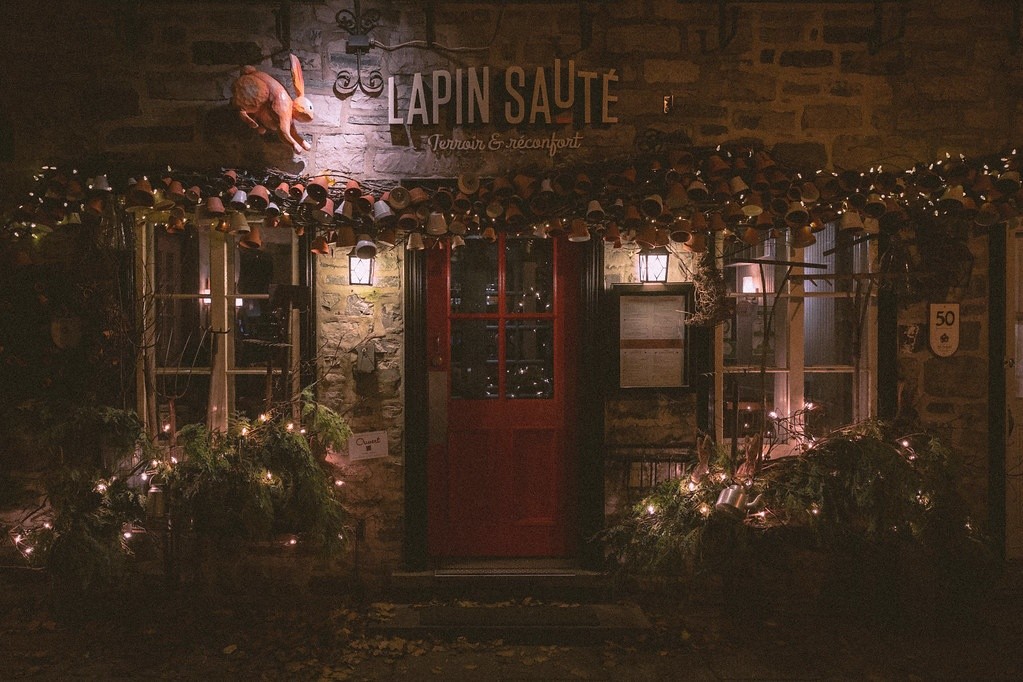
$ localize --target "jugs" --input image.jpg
[147,473,170,518]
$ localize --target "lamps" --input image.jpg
[346,247,378,286]
[636,246,672,284]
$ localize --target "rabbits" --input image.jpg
[230,52,314,155]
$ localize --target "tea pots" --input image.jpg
[716,481,762,519]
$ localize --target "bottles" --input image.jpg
[484,278,498,314]
